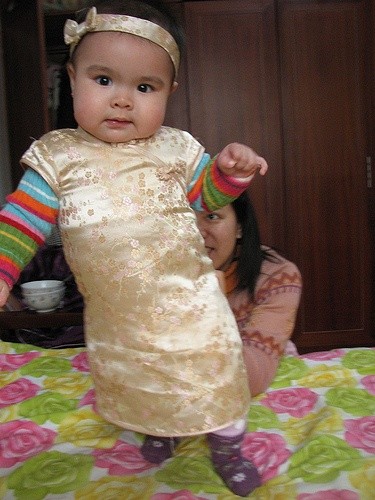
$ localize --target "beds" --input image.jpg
[0,340,375,499]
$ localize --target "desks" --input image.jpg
[0,293,84,343]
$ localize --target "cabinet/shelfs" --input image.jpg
[0,1,375,351]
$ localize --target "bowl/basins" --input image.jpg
[24,287,66,314]
[20,280,65,293]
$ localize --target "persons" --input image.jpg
[1,1,263,498]
[196,190,302,400]
